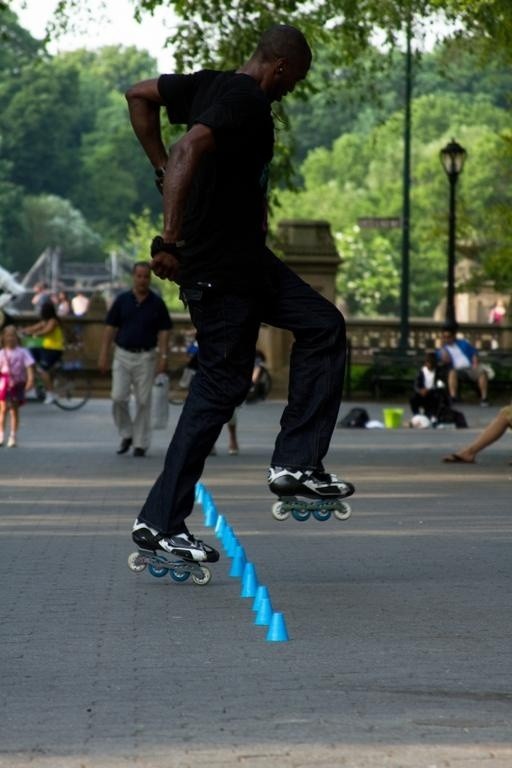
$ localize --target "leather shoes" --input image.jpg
[133,447,145,457]
[116,436,132,453]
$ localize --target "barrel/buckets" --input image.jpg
[382,407,406,429]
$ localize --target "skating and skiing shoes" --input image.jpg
[267,464,356,521]
[127,518,218,586]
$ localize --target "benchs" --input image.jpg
[368,350,497,397]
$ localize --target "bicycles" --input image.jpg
[167,360,274,406]
[16,329,94,412]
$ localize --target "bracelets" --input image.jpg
[155,166,168,191]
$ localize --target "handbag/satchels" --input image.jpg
[9,383,27,409]
[339,408,368,428]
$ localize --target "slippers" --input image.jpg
[443,453,475,463]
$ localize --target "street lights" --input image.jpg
[437,135,469,321]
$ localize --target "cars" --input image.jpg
[0,245,131,317]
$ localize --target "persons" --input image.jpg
[209,409,241,455]
[407,330,489,430]
[248,348,265,387]
[488,297,508,349]
[0,281,89,447]
[439,405,510,465]
[156,326,201,387]
[127,27,355,584]
[97,262,173,457]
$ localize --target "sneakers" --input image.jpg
[7,438,18,447]
[0,435,4,445]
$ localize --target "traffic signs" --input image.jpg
[357,217,400,229]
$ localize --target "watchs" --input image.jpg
[148,234,183,253]
